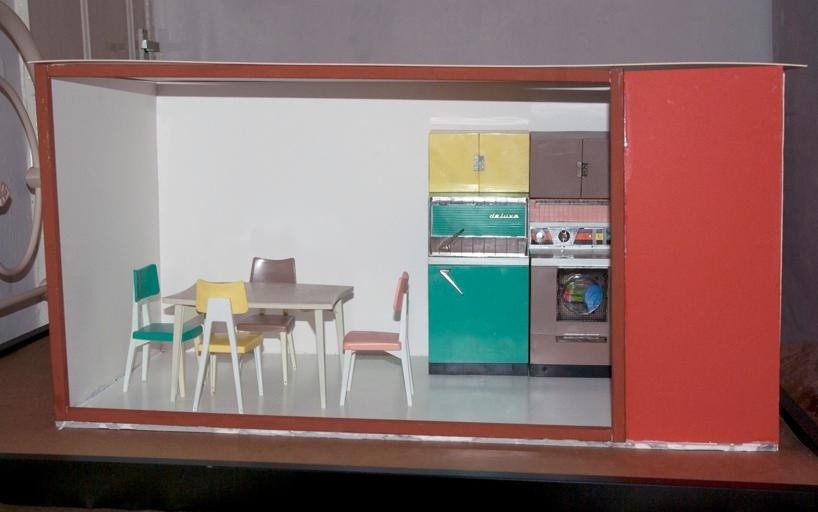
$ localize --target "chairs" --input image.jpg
[340,269,417,408]
[121,256,299,414]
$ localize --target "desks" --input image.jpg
[161,279,355,410]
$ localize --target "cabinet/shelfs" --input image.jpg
[427,130,532,195]
[528,131,610,201]
[427,265,531,376]
